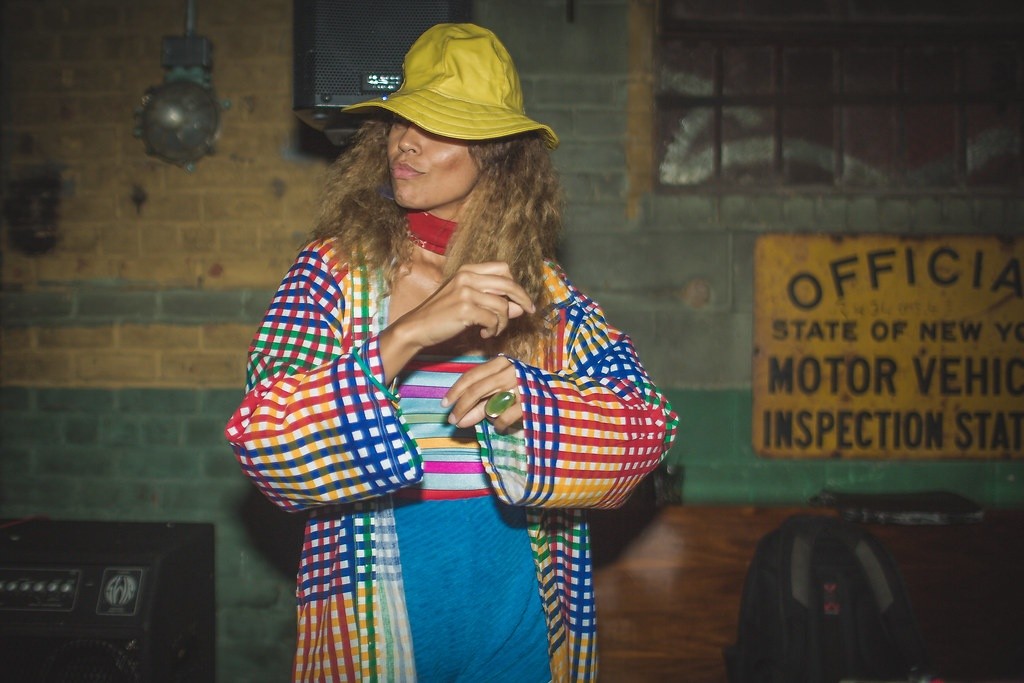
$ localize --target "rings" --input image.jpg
[484,391,515,417]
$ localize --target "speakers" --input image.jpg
[0,520,218,682]
[293,0,472,131]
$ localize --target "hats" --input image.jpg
[340,23,560,152]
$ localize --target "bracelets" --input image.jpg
[349,346,401,405]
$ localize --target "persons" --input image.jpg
[222,19,677,683]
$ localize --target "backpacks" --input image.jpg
[724,513,937,683]
